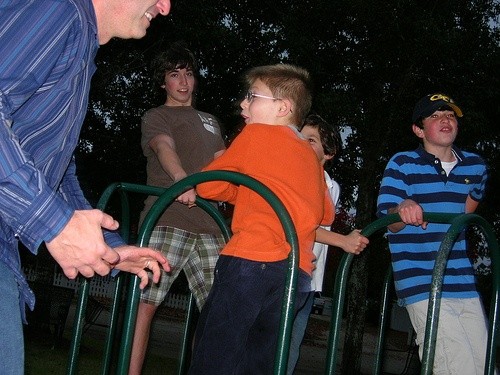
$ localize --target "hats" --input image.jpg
[412,93,463,120]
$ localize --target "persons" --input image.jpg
[127,47,226,375]
[0,0,171,375]
[376,93,488,375]
[190,64,369,375]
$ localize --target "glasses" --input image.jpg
[243,91,293,114]
